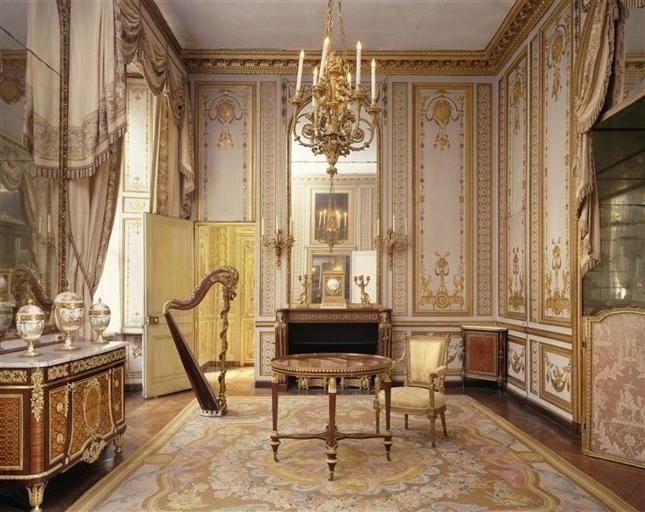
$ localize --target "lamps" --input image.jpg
[285,1,379,253]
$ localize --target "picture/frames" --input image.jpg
[306,188,356,304]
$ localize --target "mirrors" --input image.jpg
[576,89,644,316]
[1,0,70,338]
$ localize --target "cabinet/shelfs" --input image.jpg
[1,340,127,512]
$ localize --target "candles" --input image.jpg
[289,216,292,235]
[392,214,395,232]
[404,216,407,235]
[261,218,266,235]
[276,215,279,234]
[376,216,380,237]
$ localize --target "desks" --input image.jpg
[270,352,394,480]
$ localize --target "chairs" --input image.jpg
[373,330,451,448]
